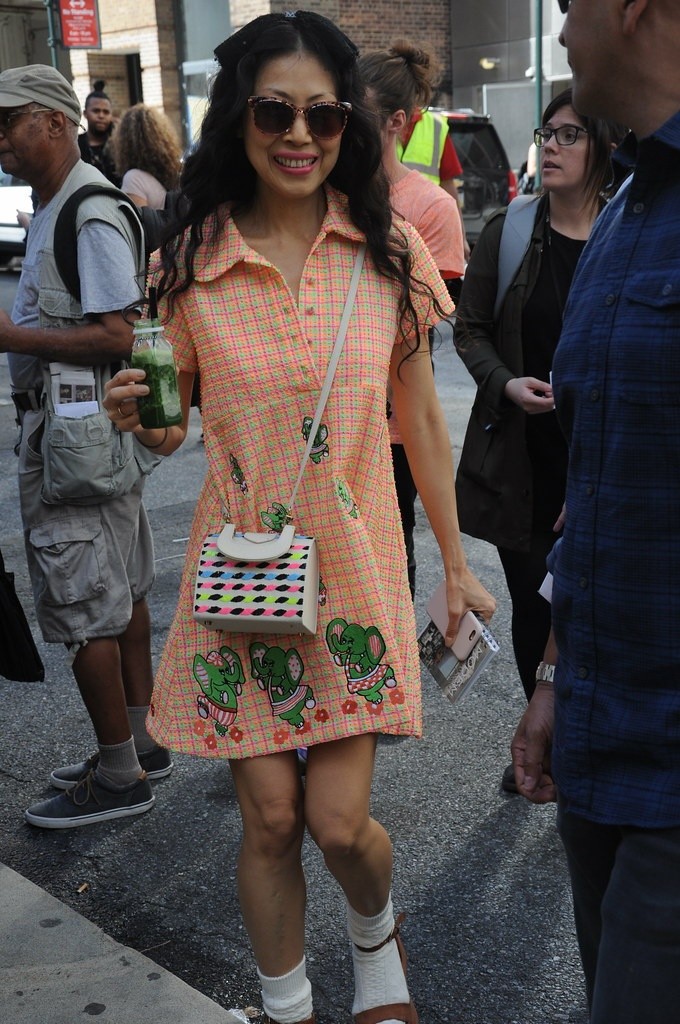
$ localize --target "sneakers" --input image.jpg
[25,769,156,829]
[50,746,174,789]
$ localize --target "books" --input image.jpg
[416,611,500,706]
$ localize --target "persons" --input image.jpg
[452,85,612,796]
[510,0,678,1024]
[77,77,205,444]
[100,9,498,1024]
[359,41,470,602]
[0,64,176,830]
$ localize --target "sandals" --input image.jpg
[262,1010,317,1024]
[351,912,419,1024]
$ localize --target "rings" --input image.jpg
[117,407,126,417]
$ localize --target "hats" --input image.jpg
[0,63,87,132]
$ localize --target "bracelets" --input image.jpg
[134,427,167,449]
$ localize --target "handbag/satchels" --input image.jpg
[193,523,320,637]
[0,548,45,682]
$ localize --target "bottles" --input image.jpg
[131,317,182,429]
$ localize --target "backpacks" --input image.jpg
[54,186,168,326]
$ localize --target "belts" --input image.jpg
[11,391,46,411]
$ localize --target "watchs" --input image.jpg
[535,662,556,682]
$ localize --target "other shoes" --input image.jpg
[502,763,519,795]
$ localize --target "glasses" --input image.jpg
[0,109,52,129]
[534,125,588,147]
[247,96,352,139]
